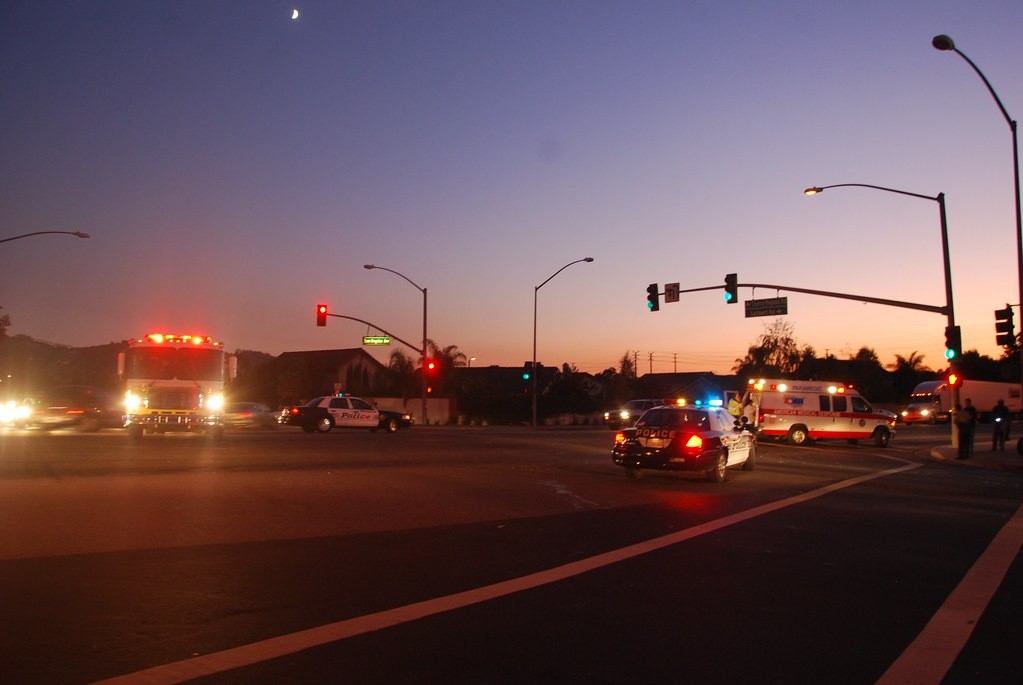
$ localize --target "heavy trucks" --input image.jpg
[901,380,1023,426]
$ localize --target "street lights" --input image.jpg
[530,257,594,427]
[363,264,427,426]
[804,183,960,451]
[933,35,1022,348]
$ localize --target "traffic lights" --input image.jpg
[425,357,436,380]
[947,372,960,386]
[646,284,659,312]
[523,362,533,383]
[994,306,1015,349]
[944,326,961,364]
[316,304,328,327]
[724,274,738,304]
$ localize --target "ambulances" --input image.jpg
[723,379,897,448]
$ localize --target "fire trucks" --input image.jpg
[117,335,237,442]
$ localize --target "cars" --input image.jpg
[222,401,278,430]
[0,381,128,437]
[278,396,415,433]
[612,406,755,488]
[604,398,666,430]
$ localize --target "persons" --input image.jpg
[728,393,756,433]
[991,399,1010,451]
[954,398,977,459]
